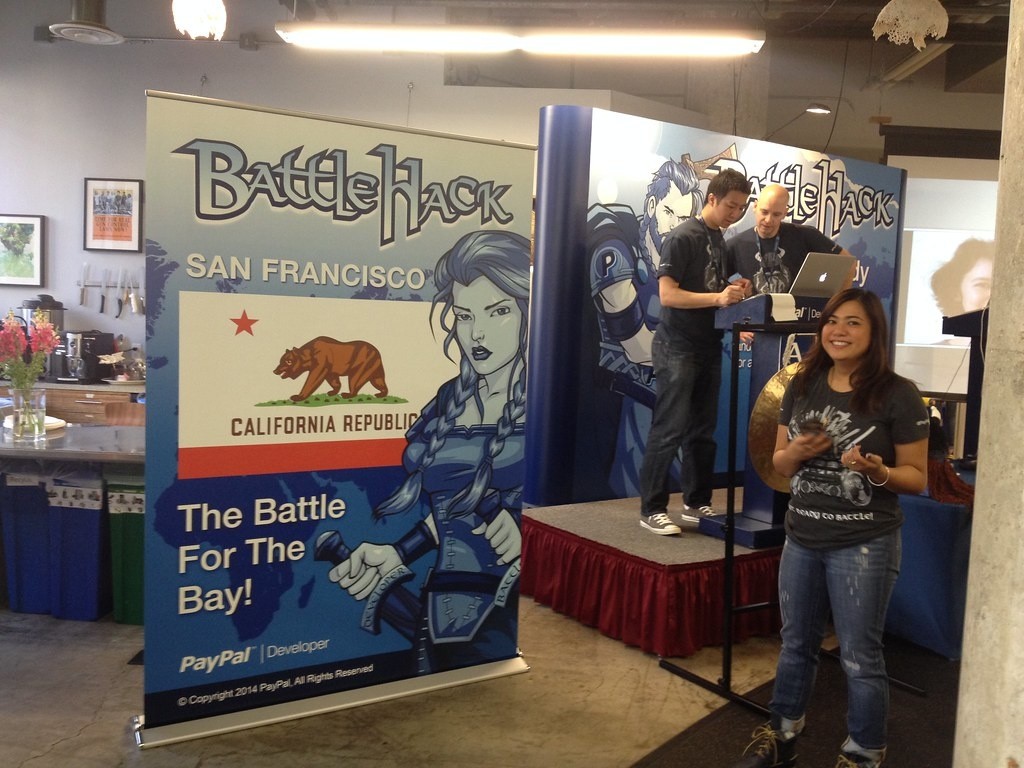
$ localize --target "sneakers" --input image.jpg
[639,513,681,535]
[682,503,717,523]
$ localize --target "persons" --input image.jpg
[726,184,858,345]
[733,288,930,768]
[640,169,751,538]
[932,237,994,348]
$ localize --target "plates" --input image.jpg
[101,376,146,386]
[2,414,66,431]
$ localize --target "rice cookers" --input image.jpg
[17,295,66,338]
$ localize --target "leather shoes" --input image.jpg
[731,721,805,768]
[832,744,887,768]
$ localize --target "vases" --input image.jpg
[13,389,46,438]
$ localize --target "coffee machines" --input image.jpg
[57,332,114,383]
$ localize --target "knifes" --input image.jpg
[115,271,123,318]
[77,263,89,305]
[99,269,107,314]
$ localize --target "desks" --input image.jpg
[0,421,145,463]
[0,380,146,426]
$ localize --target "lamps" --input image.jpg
[172,0,227,41]
[871,0,949,52]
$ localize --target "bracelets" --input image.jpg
[866,466,890,486]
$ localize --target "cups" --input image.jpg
[65,354,85,377]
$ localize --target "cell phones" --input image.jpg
[799,418,825,437]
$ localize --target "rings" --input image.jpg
[847,459,857,465]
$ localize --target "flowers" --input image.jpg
[0,304,61,436]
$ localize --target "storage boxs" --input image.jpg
[0,467,145,625]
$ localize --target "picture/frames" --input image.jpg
[83,177,143,253]
[0,214,45,288]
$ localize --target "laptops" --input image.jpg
[788,253,856,298]
